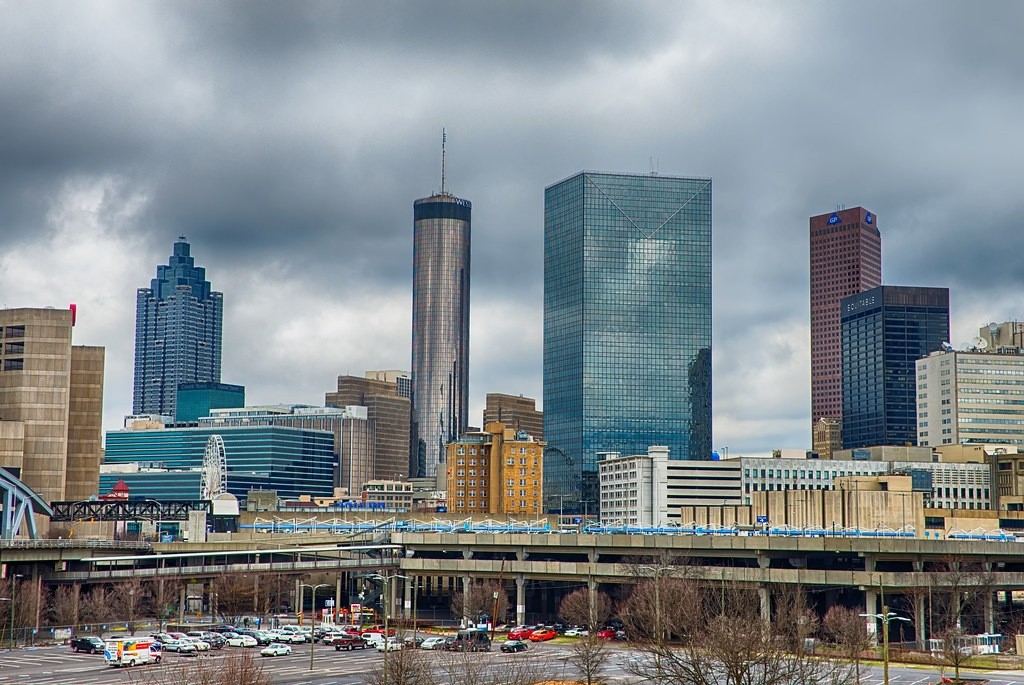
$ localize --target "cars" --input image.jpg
[500,641,528,653]
[495,624,515,633]
[510,623,588,637]
[150,624,457,657]
[529,630,557,642]
[111,635,132,638]
[597,626,628,642]
[507,629,534,640]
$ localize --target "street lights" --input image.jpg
[21,493,43,546]
[858,612,912,685]
[10,573,23,651]
[638,566,676,685]
[146,499,161,542]
[298,583,332,670]
[373,575,409,685]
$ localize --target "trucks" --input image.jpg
[104,637,161,668]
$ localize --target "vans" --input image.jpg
[457,630,492,652]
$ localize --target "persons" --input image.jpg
[244,618,251,628]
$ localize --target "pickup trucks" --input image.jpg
[71,636,105,654]
[362,625,395,637]
[333,634,368,651]
